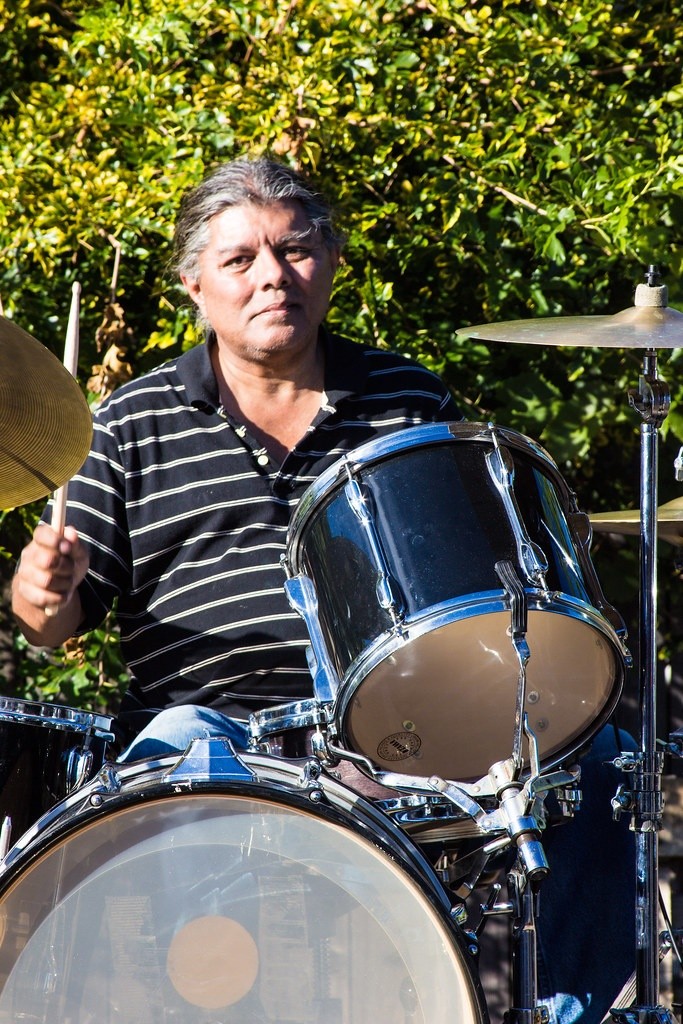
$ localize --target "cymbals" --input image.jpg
[584,497,683,544]
[455,306,683,348]
[0,314,94,507]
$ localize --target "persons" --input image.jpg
[8,150,648,1024]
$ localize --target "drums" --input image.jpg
[282,422,627,799]
[0,692,115,818]
[0,734,490,1024]
[250,701,579,844]
